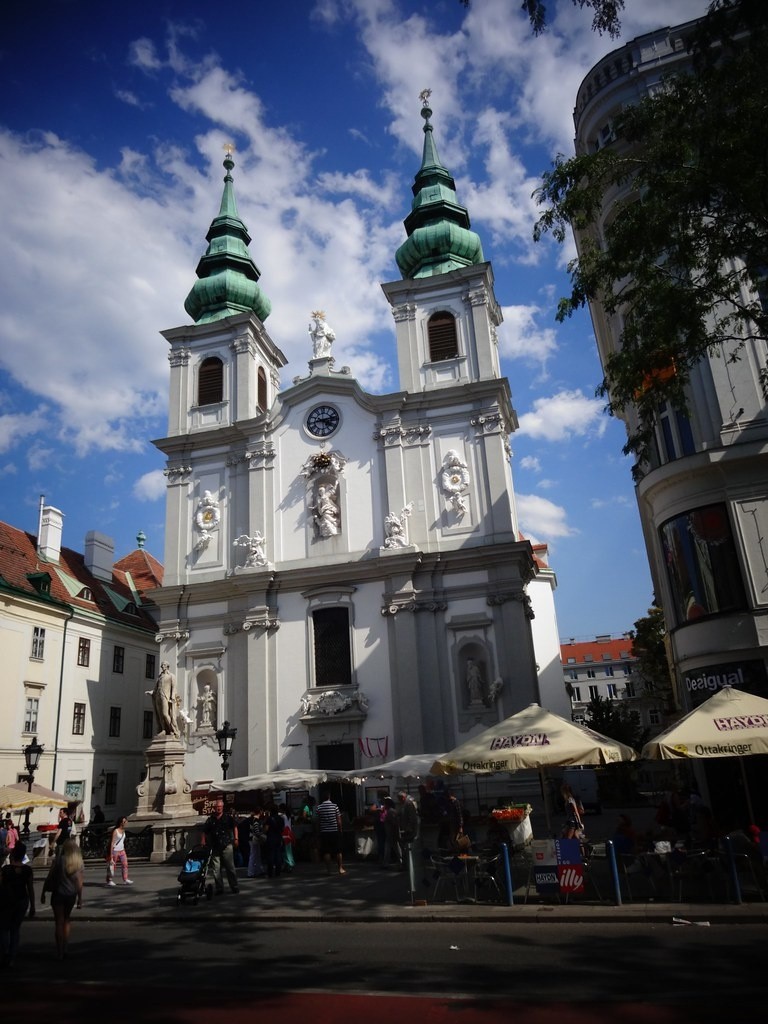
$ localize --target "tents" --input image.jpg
[343,750,448,796]
[210,768,346,815]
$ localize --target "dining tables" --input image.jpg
[429,855,471,904]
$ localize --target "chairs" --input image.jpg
[565,846,603,905]
[700,869,731,902]
[522,845,562,905]
[471,858,503,903]
[607,852,658,903]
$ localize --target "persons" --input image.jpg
[197,684,215,724]
[0,804,130,969]
[308,477,341,537]
[244,531,268,567]
[193,529,213,551]
[299,689,369,716]
[384,511,408,548]
[145,660,181,739]
[466,659,484,704]
[308,310,336,359]
[199,761,768,903]
[450,491,467,518]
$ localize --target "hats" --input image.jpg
[92,805,101,809]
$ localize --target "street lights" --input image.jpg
[214,720,240,805]
[19,738,44,832]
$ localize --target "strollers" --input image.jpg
[176,843,217,906]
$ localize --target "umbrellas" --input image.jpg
[431,701,642,830]
[0,780,77,814]
[640,685,768,823]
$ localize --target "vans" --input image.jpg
[558,770,604,817]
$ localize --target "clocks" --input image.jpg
[302,402,343,441]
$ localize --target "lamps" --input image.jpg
[92,769,107,795]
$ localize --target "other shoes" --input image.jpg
[53,952,64,960]
[65,948,72,957]
[1,958,14,968]
[107,880,116,886]
[123,879,132,885]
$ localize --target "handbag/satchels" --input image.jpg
[253,835,267,844]
[106,840,115,857]
[107,858,114,877]
[575,800,584,814]
[454,832,472,849]
[22,854,30,864]
[46,855,63,891]
